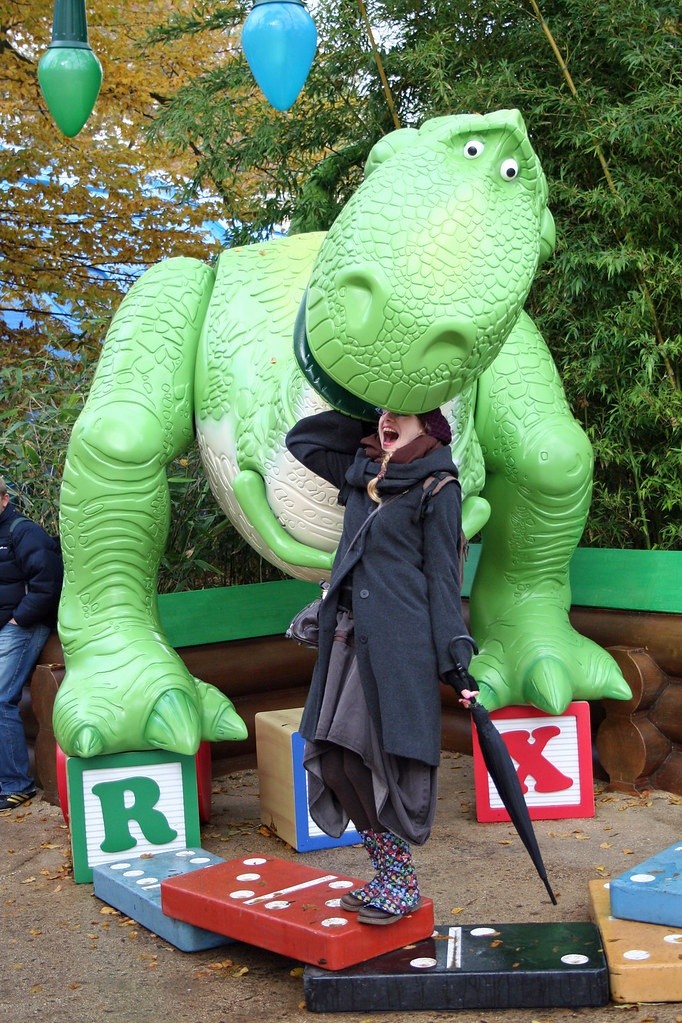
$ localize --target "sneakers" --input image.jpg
[0,787,36,810]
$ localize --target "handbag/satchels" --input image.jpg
[286,598,321,651]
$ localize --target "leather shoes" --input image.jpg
[357,892,422,925]
[340,894,367,912]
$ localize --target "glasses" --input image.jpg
[375,408,412,419]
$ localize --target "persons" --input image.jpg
[0,476,64,809]
[285,394,475,923]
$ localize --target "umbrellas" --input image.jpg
[449,635,557,906]
